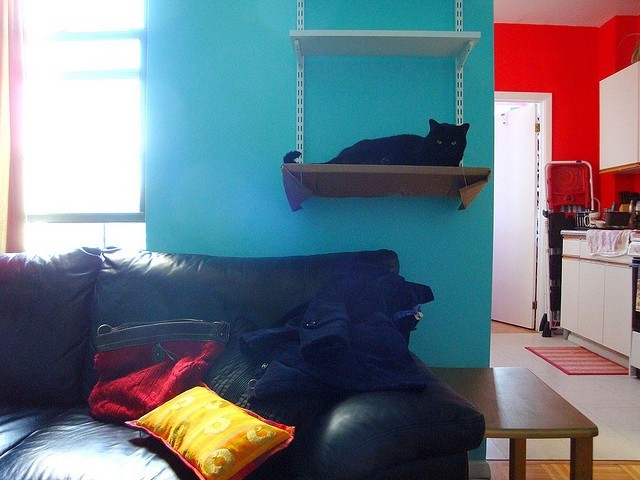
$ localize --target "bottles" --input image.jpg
[628,200,634,224]
[611,202,616,212]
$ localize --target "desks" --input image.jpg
[418,365,599,480]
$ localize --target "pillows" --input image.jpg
[202,315,294,427]
[124,383,295,480]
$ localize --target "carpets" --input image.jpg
[526,346,630,375]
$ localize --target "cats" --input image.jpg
[281,117,473,167]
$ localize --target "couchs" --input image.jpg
[2,249,488,480]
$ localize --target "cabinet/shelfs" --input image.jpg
[542,161,594,336]
[599,62,640,172]
[559,229,605,354]
[281,32,492,210]
[605,228,640,374]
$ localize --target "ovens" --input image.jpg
[627,242,640,376]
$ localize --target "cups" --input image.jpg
[596,221,605,228]
[583,212,598,228]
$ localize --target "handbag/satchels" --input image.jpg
[202,314,336,429]
[88,321,230,430]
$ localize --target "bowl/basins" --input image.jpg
[605,212,633,226]
